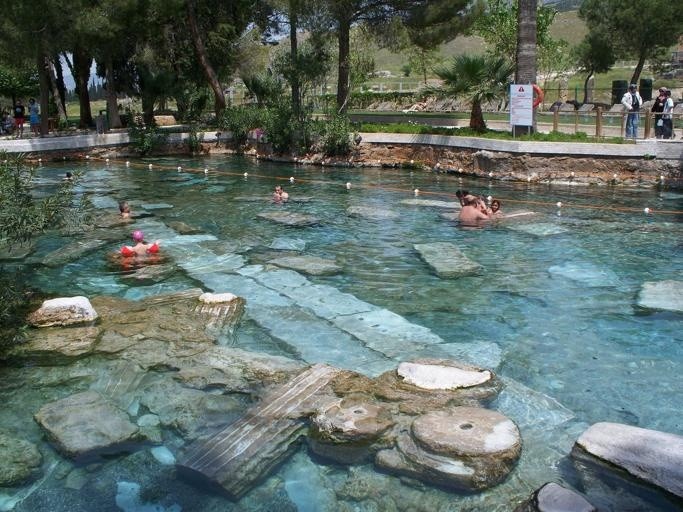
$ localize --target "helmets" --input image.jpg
[132,230,144,242]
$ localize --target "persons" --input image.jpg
[272,185,288,200]
[0,112,14,135]
[456,190,500,225]
[661,91,674,139]
[649,87,676,140]
[62,172,73,180]
[118,201,130,218]
[126,230,159,255]
[620,85,644,138]
[27,99,38,136]
[13,99,25,138]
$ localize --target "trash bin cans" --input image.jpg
[612,80,628,104]
[639,78,652,101]
[96,110,111,134]
[48,115,60,132]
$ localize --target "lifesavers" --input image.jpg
[532,84,543,109]
